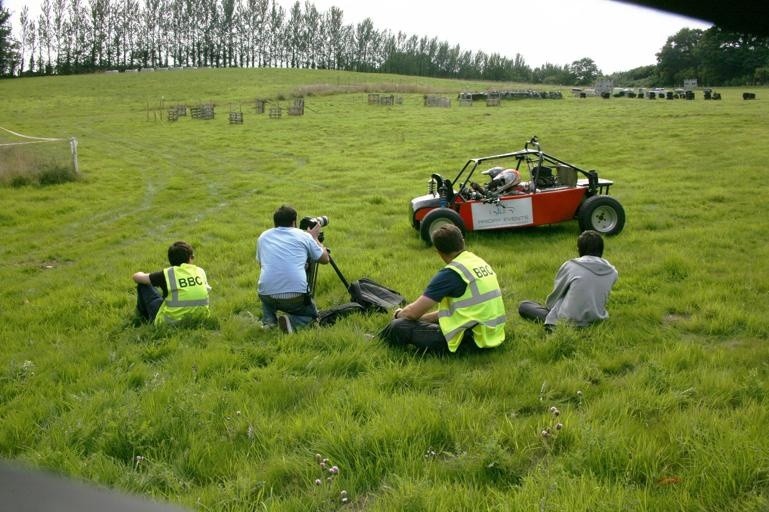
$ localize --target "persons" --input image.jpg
[517,229,619,332]
[364,223,507,356]
[132,241,212,325]
[481,166,506,192]
[253,204,330,334]
[470,169,527,201]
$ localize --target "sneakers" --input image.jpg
[279,315,292,335]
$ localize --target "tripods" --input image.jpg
[310,256,350,297]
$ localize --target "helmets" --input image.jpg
[493,168,522,188]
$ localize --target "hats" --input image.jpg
[482,166,504,177]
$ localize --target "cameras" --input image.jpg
[299,215,329,232]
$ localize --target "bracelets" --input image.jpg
[394,309,401,319]
